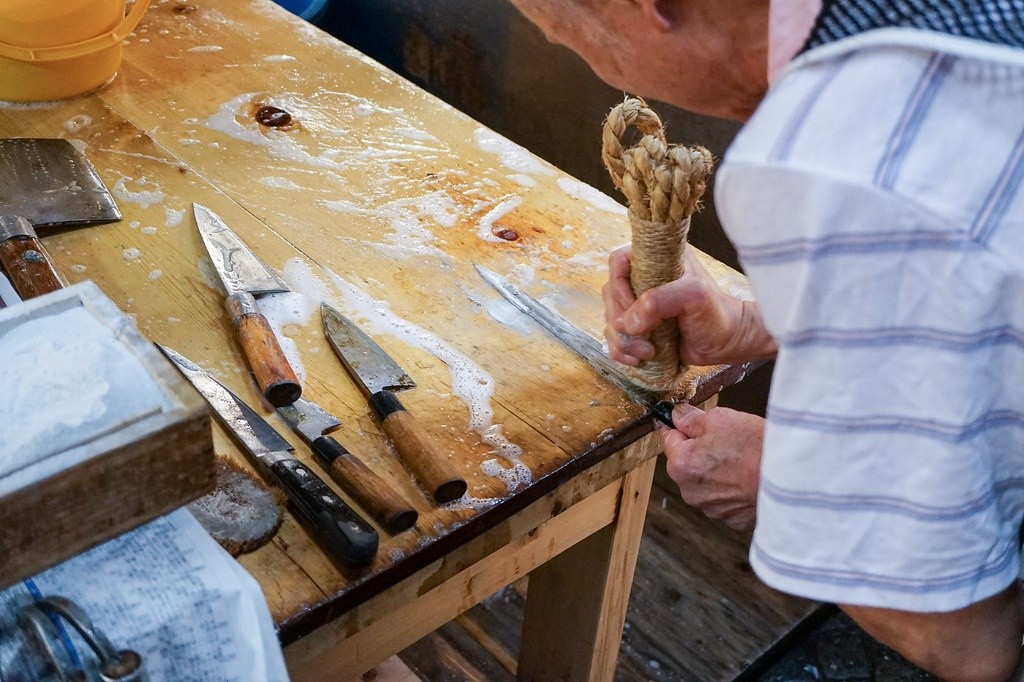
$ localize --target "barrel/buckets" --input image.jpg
[0,1,152,102]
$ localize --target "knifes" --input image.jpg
[474,262,687,434]
[192,201,301,406]
[320,302,467,504]
[266,396,418,533]
[0,138,123,301]
[152,340,379,570]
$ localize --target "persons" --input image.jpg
[511,2,1024,682]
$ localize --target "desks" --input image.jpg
[1,0,770,682]
